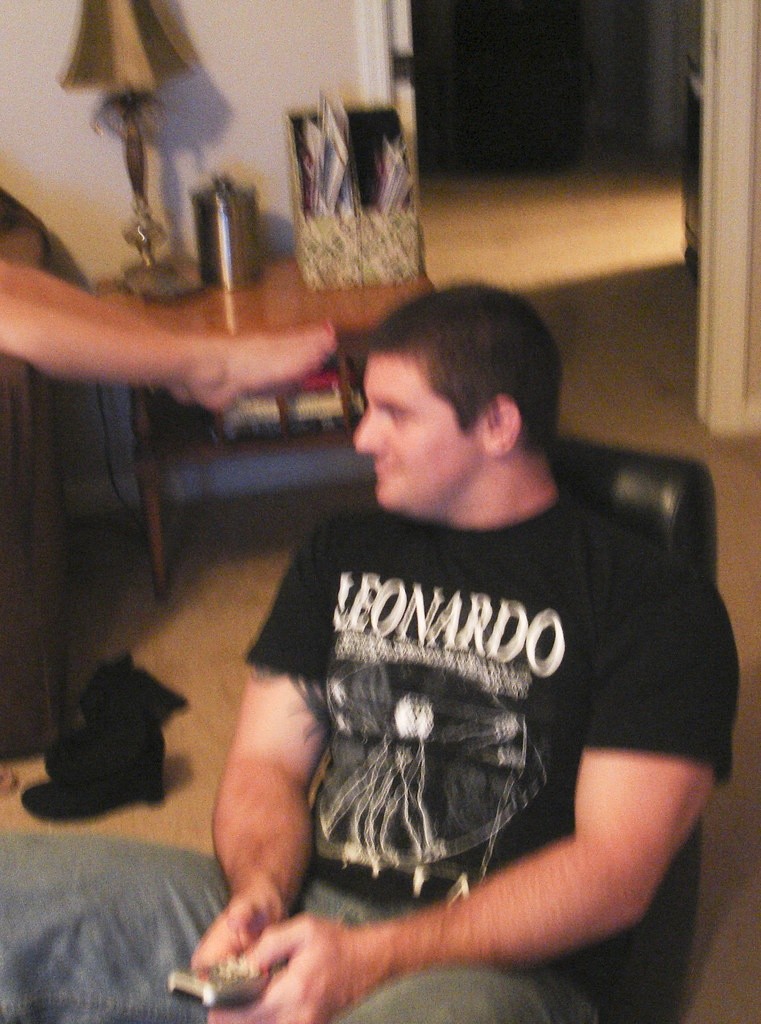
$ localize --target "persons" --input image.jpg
[0,249,347,419]
[0,286,737,1023]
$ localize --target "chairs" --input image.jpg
[552,445,717,1023]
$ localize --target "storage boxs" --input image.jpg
[282,83,423,292]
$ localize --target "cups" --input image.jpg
[191,179,265,293]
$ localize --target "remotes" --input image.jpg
[168,964,271,1008]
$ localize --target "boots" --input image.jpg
[21,648,187,820]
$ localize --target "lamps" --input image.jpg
[60,0,201,304]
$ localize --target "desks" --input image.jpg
[98,254,435,598]
